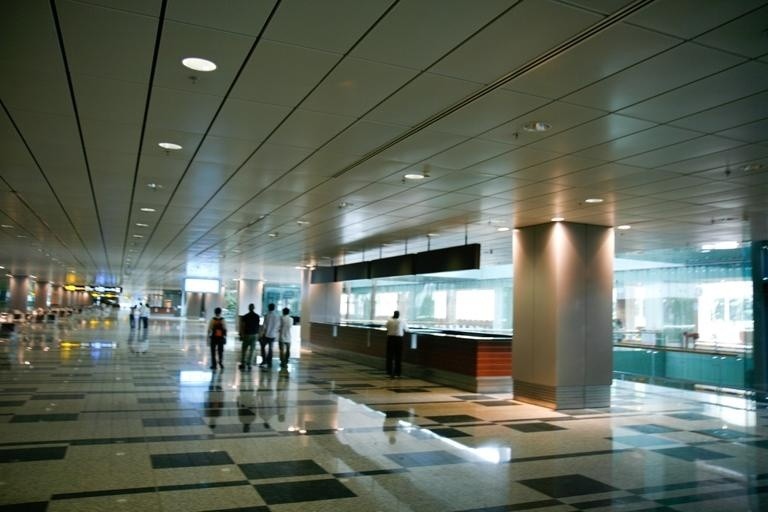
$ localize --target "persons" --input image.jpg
[237,301,260,371]
[278,307,295,376]
[206,306,226,372]
[137,301,144,331]
[128,329,150,356]
[129,304,138,330]
[142,303,151,330]
[257,302,281,368]
[383,310,410,378]
[205,367,291,432]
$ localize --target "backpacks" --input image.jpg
[212,317,224,342]
